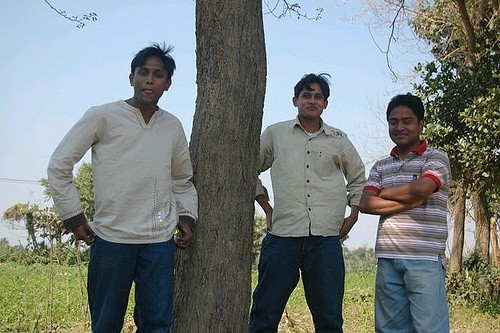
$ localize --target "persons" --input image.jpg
[249,73,366,333]
[359,95,452,333]
[47,41,198,333]
